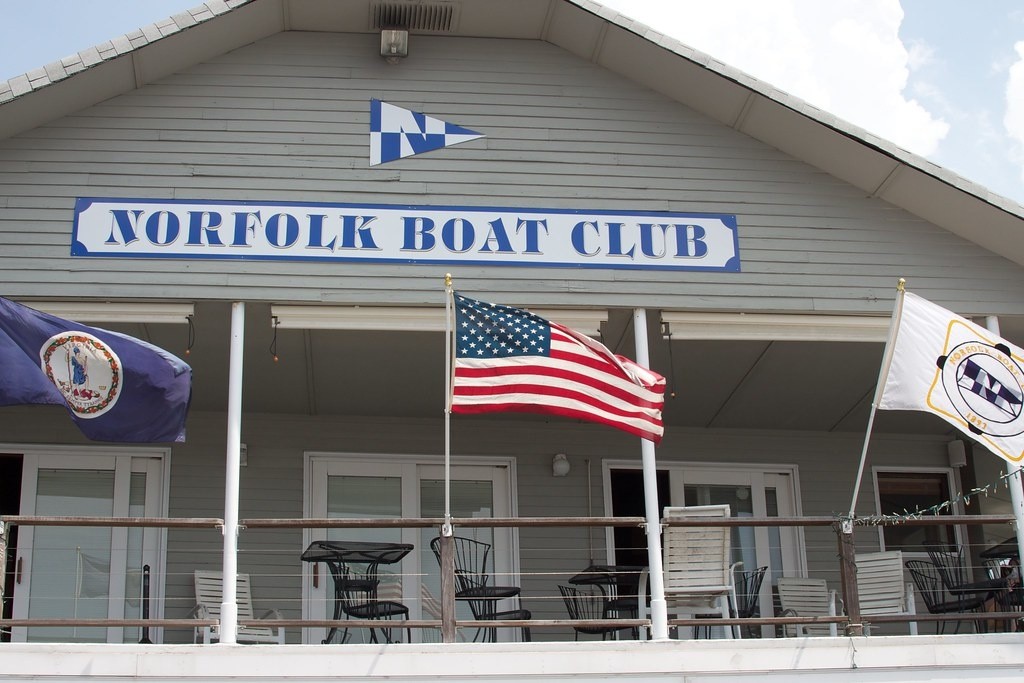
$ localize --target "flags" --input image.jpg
[78,554,140,607]
[877,292,1024,467]
[349,563,442,619]
[450,291,666,445]
[0,296,194,445]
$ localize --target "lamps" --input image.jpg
[380,25,408,65]
[552,454,571,477]
[949,440,967,468]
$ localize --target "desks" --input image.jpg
[300,540,414,644]
[981,536,1024,631]
[568,565,651,640]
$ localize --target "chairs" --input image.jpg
[695,566,768,639]
[597,584,638,640]
[905,540,1024,634]
[431,535,532,643]
[337,566,412,644]
[777,577,847,637]
[194,570,285,645]
[558,585,639,641]
[828,549,918,636]
[638,504,744,639]
